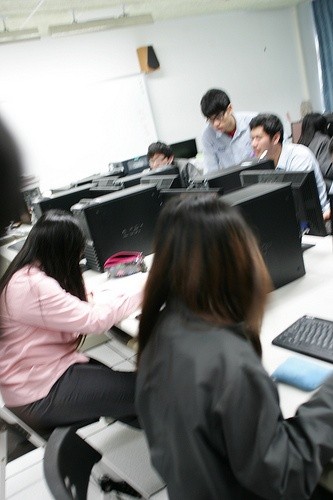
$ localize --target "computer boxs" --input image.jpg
[110,155,150,173]
[220,182,306,292]
[70,185,161,273]
[169,139,198,158]
[206,158,275,195]
[32,183,108,223]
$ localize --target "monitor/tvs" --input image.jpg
[120,167,182,189]
[140,174,181,190]
[240,170,325,237]
[160,186,224,206]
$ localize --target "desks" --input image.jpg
[0,221,333,419]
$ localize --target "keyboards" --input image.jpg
[273,315,333,362]
[9,239,25,251]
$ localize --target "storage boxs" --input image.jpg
[290,121,302,144]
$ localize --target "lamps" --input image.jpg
[49,1,153,39]
[0,14,40,43]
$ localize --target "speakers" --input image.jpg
[135,45,160,74]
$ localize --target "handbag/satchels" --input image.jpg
[104,249,146,279]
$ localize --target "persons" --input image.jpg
[201,88,260,174]
[143,141,202,189]
[297,112,333,193]
[249,113,332,235]
[0,209,149,429]
[134,194,333,500]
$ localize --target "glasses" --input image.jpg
[149,156,167,167]
[205,106,226,125]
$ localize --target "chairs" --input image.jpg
[0,392,152,500]
